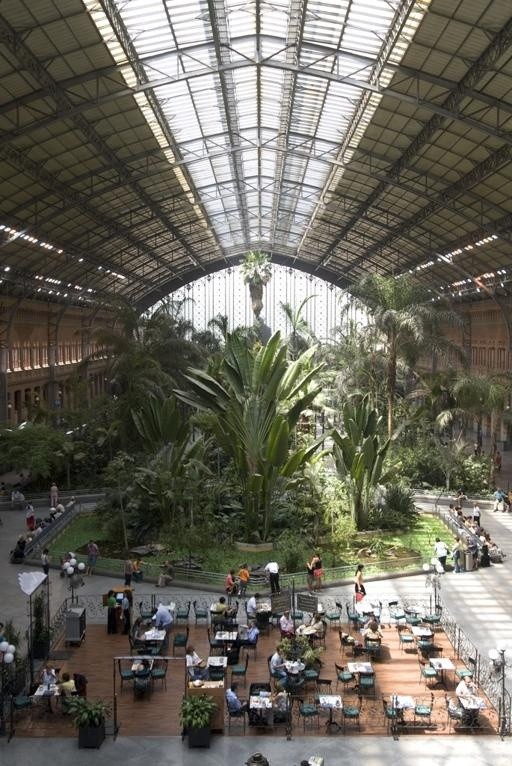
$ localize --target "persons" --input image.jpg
[215,596,233,618]
[265,559,280,592]
[496,451,502,472]
[40,663,79,715]
[306,553,322,591]
[363,624,382,655]
[232,620,259,658]
[270,648,289,724]
[185,646,209,681]
[433,502,507,574]
[507,489,512,512]
[84,540,98,576]
[354,565,366,597]
[308,614,323,646]
[155,560,174,587]
[107,590,133,635]
[135,659,150,689]
[455,676,480,727]
[473,440,481,456]
[457,486,470,509]
[247,592,262,617]
[11,483,74,562]
[152,608,174,648]
[226,682,254,725]
[226,563,250,596]
[134,619,146,644]
[493,487,508,512]
[41,548,50,585]
[125,555,143,587]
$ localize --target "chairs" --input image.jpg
[176,692,219,749]
[65,693,112,749]
[17,601,477,735]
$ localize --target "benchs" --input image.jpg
[448,509,502,563]
[11,500,78,564]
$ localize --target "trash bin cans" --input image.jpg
[464,551,473,571]
